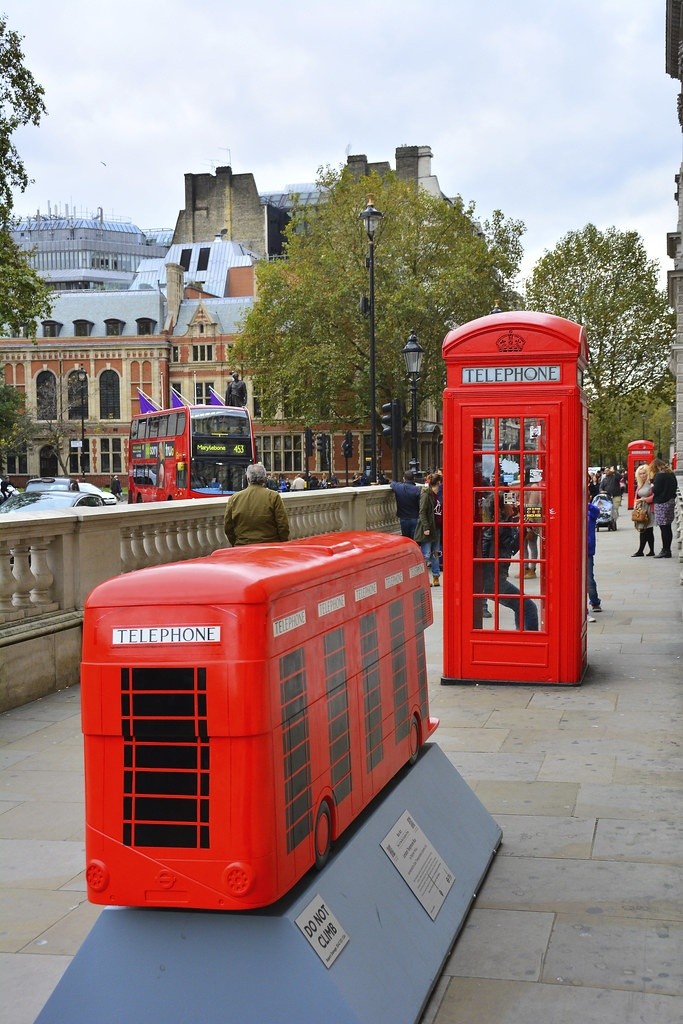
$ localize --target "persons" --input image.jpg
[389,470,423,546]
[648,458,677,558]
[630,465,656,556]
[351,470,390,487]
[1,476,12,502]
[224,462,290,547]
[110,475,124,501]
[225,373,247,407]
[480,493,539,634]
[265,472,337,493]
[417,465,627,617]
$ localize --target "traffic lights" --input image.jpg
[382,401,394,438]
[344,432,354,458]
[316,434,326,452]
[304,431,314,457]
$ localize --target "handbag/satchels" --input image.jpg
[632,503,648,522]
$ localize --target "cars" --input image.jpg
[0,490,106,572]
[0,479,20,497]
[76,483,117,505]
[24,477,81,492]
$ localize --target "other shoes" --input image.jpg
[632,552,644,557]
[646,552,655,556]
[591,604,602,612]
[586,615,596,622]
[482,610,491,618]
[654,551,671,558]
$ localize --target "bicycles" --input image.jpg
[0,490,18,505]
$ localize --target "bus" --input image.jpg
[79,531,440,910]
[127,405,256,504]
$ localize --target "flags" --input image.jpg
[138,391,157,415]
[209,390,223,405]
[172,389,184,408]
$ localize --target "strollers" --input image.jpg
[591,492,617,531]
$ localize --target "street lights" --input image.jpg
[401,328,426,483]
[76,363,88,482]
[357,193,383,486]
[641,412,647,439]
[657,423,663,458]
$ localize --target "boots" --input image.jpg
[524,568,536,579]
[515,568,529,578]
[434,576,440,586]
